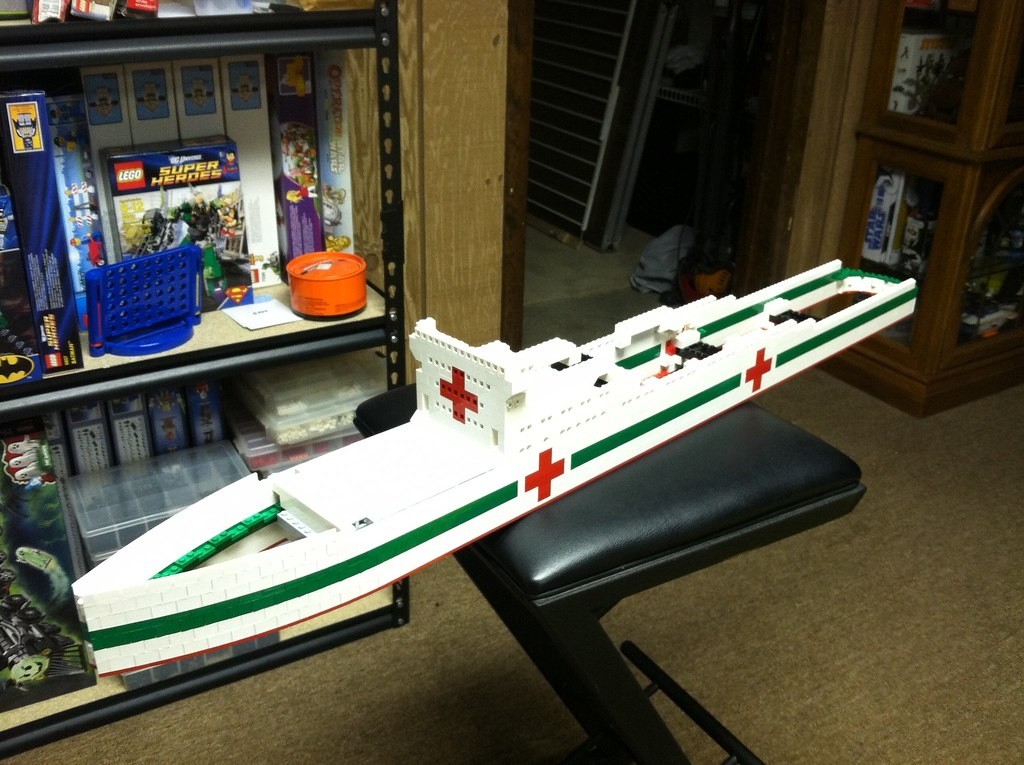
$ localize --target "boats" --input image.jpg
[71,258,917,680]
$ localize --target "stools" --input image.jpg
[353,381,867,762]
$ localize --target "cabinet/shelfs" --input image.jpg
[812,0,1024,416]
[0,0,413,759]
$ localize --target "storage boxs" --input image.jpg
[1,46,394,695]
